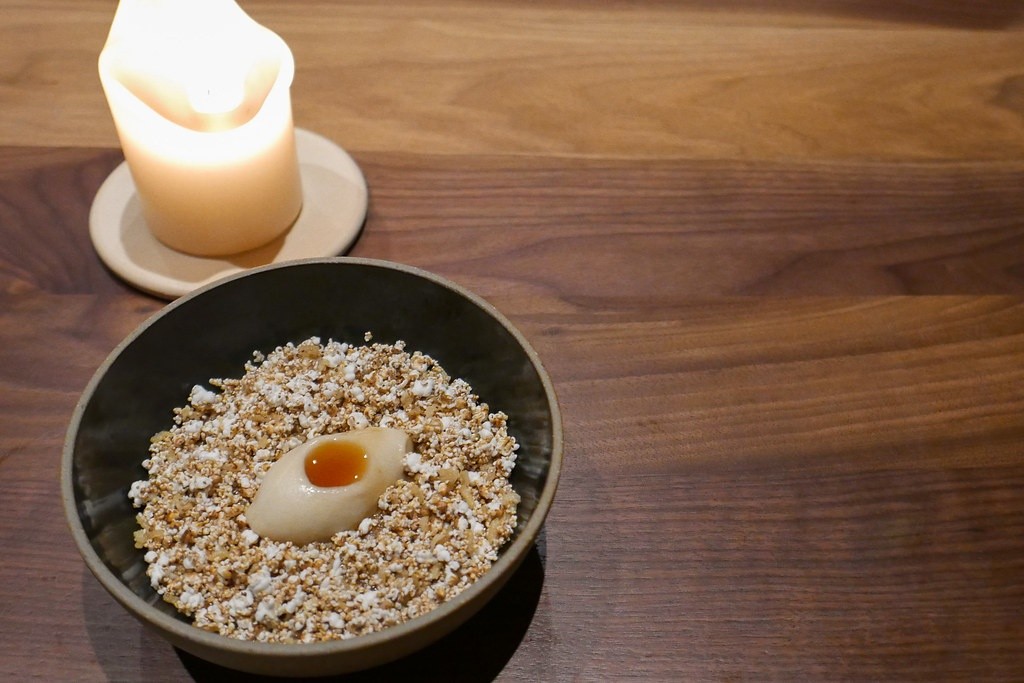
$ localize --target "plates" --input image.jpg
[89,131,368,297]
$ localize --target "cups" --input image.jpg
[97,0,303,256]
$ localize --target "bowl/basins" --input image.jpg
[59,257,562,680]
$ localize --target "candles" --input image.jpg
[97,0,304,257]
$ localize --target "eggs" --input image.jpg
[245,428,414,546]
